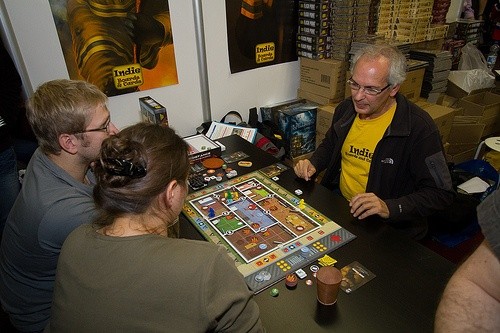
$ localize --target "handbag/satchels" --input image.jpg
[427,159,500,248]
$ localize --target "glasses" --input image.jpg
[59,118,110,134]
[346,76,391,96]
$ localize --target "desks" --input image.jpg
[167,135,460,333]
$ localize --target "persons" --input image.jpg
[434,189,500,333]
[44,122,267,333]
[293,43,454,241]
[0,79,121,333]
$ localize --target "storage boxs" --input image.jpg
[139,0,500,233]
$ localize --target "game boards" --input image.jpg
[181,170,357,294]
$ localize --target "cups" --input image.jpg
[316,267,342,305]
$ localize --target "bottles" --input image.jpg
[486,41,499,71]
